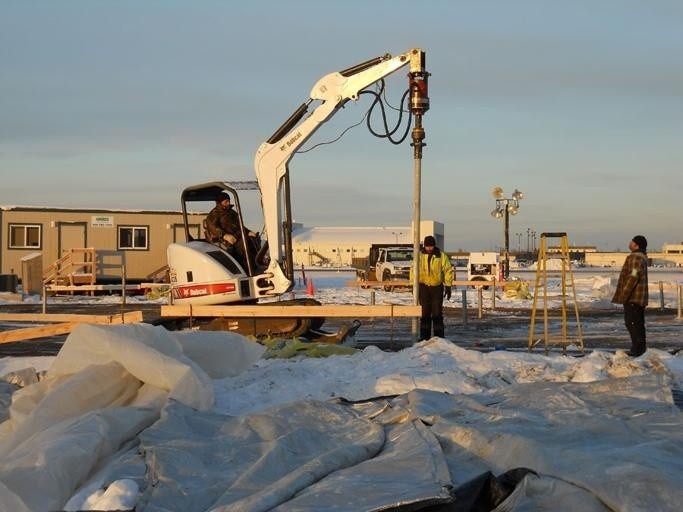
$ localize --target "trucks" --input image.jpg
[350,243,423,292]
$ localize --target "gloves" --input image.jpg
[444,286,451,300]
[248,232,256,237]
[223,234,236,245]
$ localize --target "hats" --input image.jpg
[215,192,230,204]
[424,236,435,246]
[632,236,647,246]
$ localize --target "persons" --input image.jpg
[205,190,268,295]
[608,234,649,356]
[408,235,453,342]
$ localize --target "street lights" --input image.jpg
[489,185,524,280]
[515,228,536,253]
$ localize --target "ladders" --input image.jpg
[528,233,585,357]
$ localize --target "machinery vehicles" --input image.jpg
[164,39,429,343]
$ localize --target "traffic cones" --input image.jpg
[305,277,314,295]
